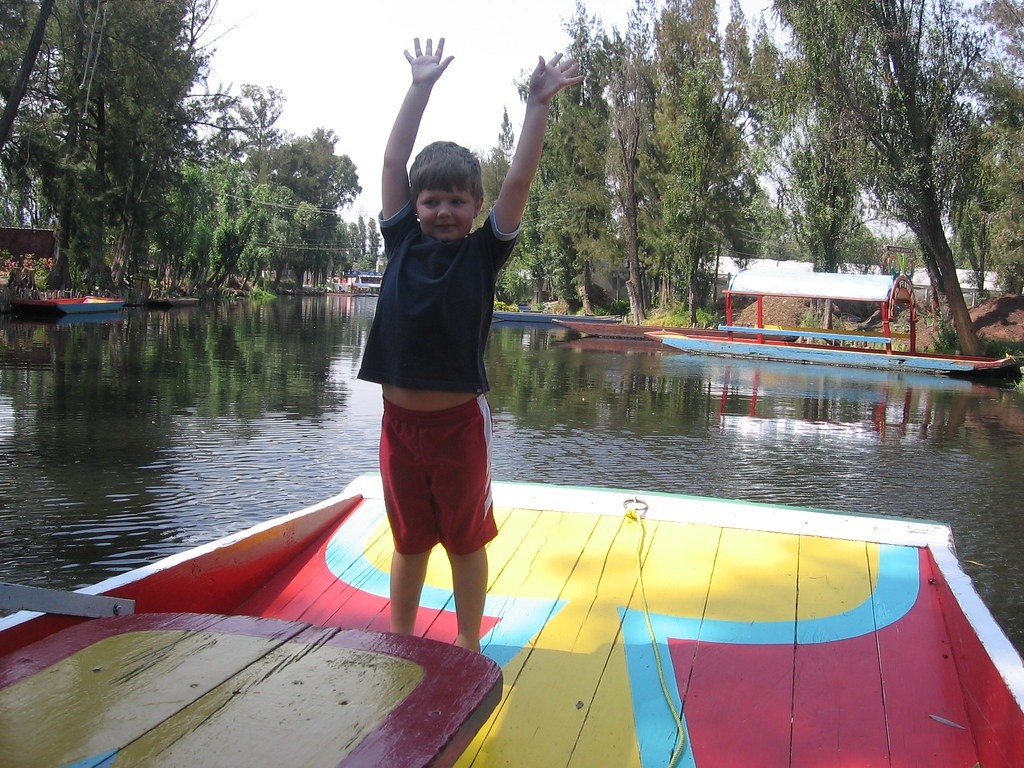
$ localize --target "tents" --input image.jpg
[703,254,1006,306]
[343,270,384,284]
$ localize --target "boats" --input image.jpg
[644,265,1019,376]
[552,317,800,342]
[167,297,203,307]
[10,295,125,316]
[493,310,622,324]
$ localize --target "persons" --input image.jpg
[227,274,253,291]
[357,34,588,654]
